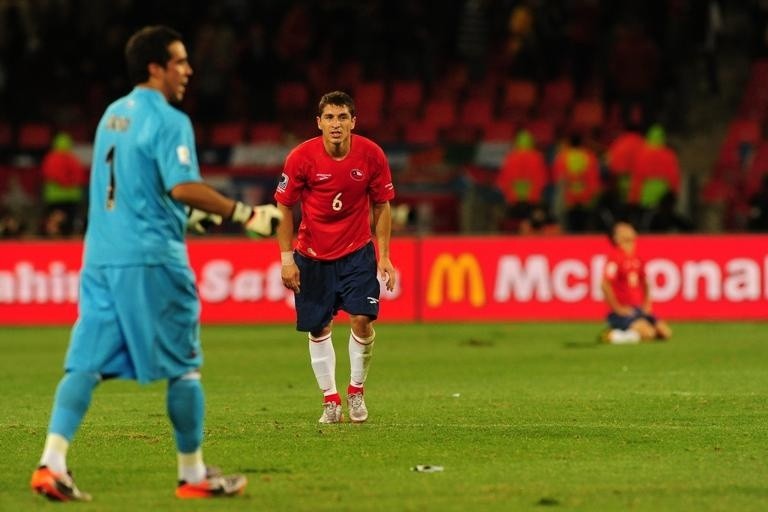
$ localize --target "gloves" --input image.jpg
[230,202,282,237]
[185,206,223,232]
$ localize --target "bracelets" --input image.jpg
[281,251,295,266]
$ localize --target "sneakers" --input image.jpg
[32,464,90,503]
[347,393,368,422]
[319,402,345,422]
[176,465,247,497]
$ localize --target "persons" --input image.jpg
[270,91,400,427]
[31,25,287,505]
[486,1,768,238]
[596,221,673,344]
[0,176,35,239]
[39,133,87,240]
